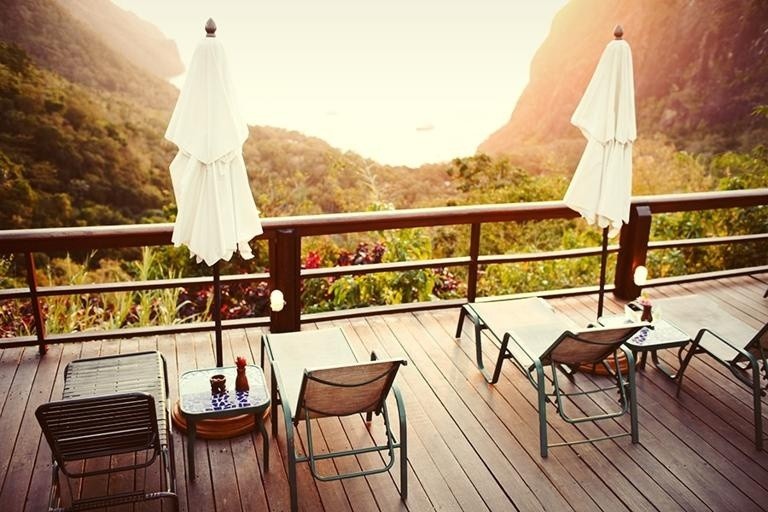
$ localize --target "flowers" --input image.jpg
[234,355,247,368]
[637,291,652,306]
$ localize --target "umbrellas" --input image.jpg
[164,14,264,369]
[562,22,636,325]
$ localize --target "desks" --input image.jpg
[177,364,271,486]
[597,310,691,402]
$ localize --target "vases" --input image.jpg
[236,367,249,392]
[641,305,652,322]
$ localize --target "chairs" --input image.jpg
[627,293,768,452]
[34,351,181,511]
[455,297,654,458]
[259,326,408,512]
